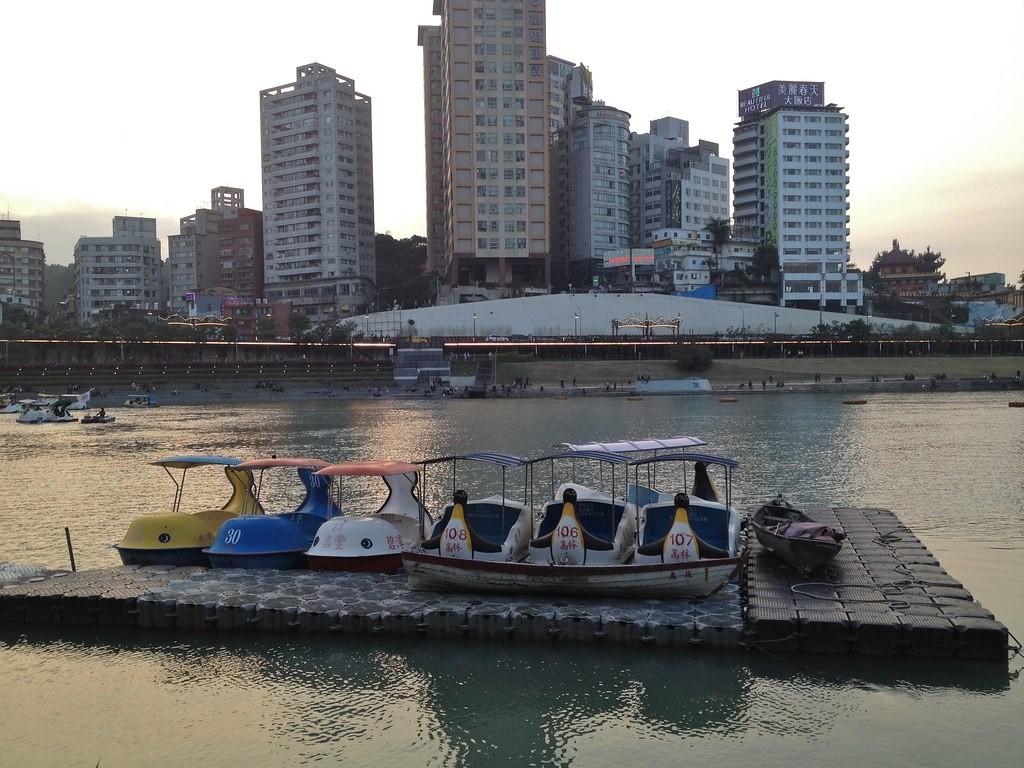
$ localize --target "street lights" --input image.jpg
[473,313,477,341]
[574,313,579,338]
[677,313,681,335]
[365,315,369,335]
[774,313,779,332]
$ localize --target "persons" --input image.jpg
[344,384,352,390]
[129,380,157,392]
[984,369,1020,390]
[540,385,543,390]
[482,375,530,393]
[65,383,81,394]
[606,380,631,392]
[257,377,277,392]
[172,388,177,394]
[581,388,585,394]
[870,376,884,381]
[637,374,650,382]
[739,376,783,390]
[425,375,458,396]
[905,374,946,388]
[406,385,415,391]
[197,383,202,388]
[814,373,842,382]
[367,385,388,396]
[13,386,23,392]
[464,383,471,390]
[572,376,577,388]
[97,408,105,417]
[560,376,565,388]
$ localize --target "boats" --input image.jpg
[37,391,91,410]
[81,415,115,424]
[0,402,28,413]
[402,549,751,600]
[113,456,265,567]
[202,459,345,571]
[625,451,746,566]
[304,459,431,574]
[752,493,843,572]
[124,395,158,408]
[530,436,658,567]
[416,450,535,563]
[16,407,79,424]
